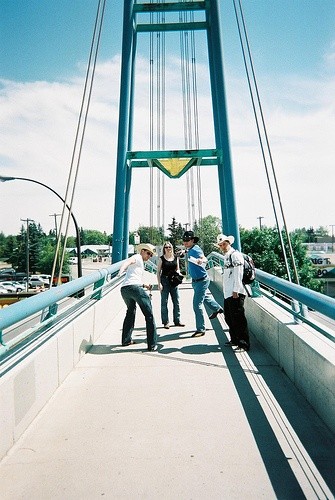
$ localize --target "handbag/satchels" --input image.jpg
[168,272,183,288]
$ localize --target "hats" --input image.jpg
[215,234,234,245]
[180,231,199,243]
[137,243,158,257]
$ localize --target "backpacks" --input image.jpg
[229,250,256,284]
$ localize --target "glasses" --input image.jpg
[164,247,171,248]
[183,239,190,242]
[145,251,152,257]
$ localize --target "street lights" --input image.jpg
[0,174,86,299]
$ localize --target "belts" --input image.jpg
[192,275,207,282]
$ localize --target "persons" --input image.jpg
[157,242,185,328]
[216,234,249,352]
[180,231,223,336]
[116,244,160,351]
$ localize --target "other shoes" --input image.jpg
[122,341,135,346]
[164,323,169,329]
[149,345,158,351]
[209,308,224,319]
[224,341,237,346]
[191,332,205,337]
[233,346,248,352]
[175,323,185,327]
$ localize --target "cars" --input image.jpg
[31,274,54,288]
[0,281,17,293]
[22,277,45,289]
[8,281,26,292]
[66,256,78,265]
[0,285,8,294]
